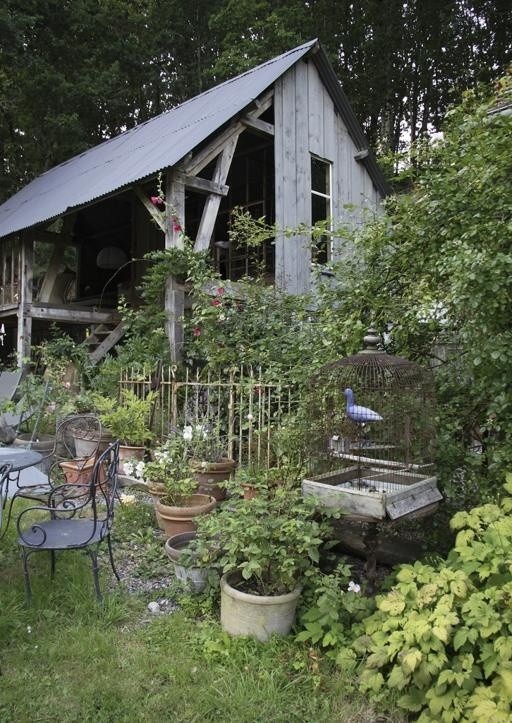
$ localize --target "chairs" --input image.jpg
[0,416,102,540]
[16,437,122,603]
[0,463,13,533]
[0,392,38,436]
[0,371,23,401]
[60,272,76,304]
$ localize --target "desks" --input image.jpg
[0,446,44,510]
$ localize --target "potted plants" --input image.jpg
[139,384,346,642]
[56,384,162,462]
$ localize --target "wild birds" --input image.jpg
[338,388,384,428]
